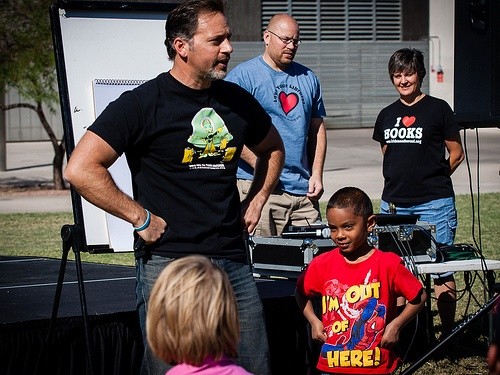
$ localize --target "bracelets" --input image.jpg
[132,208,151,231]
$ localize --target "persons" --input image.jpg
[145,254,257,375]
[224,13,327,237]
[293,187,426,375]
[63,0,286,375]
[373,47,465,337]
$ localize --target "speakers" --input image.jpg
[452,0,500,131]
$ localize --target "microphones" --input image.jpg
[281,227,332,239]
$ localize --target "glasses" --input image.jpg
[267,29,302,47]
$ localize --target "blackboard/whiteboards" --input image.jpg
[50,5,174,251]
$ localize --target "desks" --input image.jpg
[253,258,500,355]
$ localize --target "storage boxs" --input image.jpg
[250,236,372,274]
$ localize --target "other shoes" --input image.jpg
[441,331,456,341]
